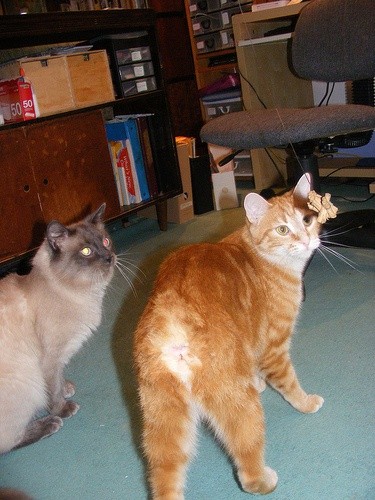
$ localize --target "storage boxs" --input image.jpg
[108,29,159,97]
[1,49,117,120]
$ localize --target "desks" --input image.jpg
[230,1,323,200]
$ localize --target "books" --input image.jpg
[105,111,175,208]
[0,0,153,13]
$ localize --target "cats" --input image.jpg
[131,173,364,499]
[0,202,148,455]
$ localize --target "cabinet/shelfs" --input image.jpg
[0,88,185,278]
[188,0,254,209]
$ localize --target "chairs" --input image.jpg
[196,0,375,252]
